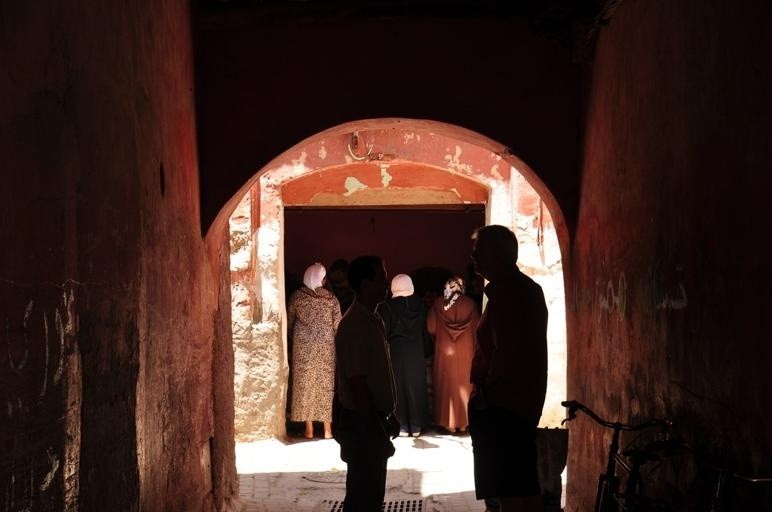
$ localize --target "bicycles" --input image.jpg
[561,399,771,511]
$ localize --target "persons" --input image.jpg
[468,225,548,512]
[286,258,480,440]
[332,255,401,512]
